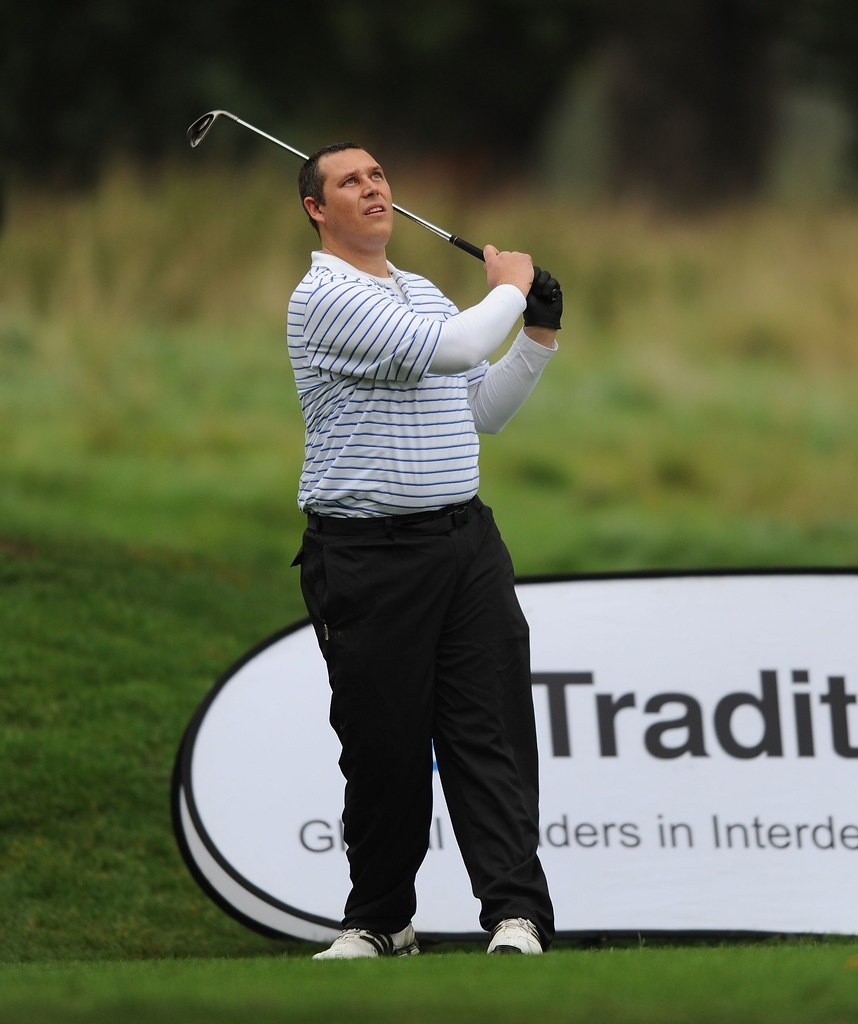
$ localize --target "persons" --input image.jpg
[286,145,564,960]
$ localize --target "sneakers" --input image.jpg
[312,922,420,959]
[487,918,543,957]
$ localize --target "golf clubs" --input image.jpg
[189,109,485,260]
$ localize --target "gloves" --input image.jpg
[522,266,563,329]
